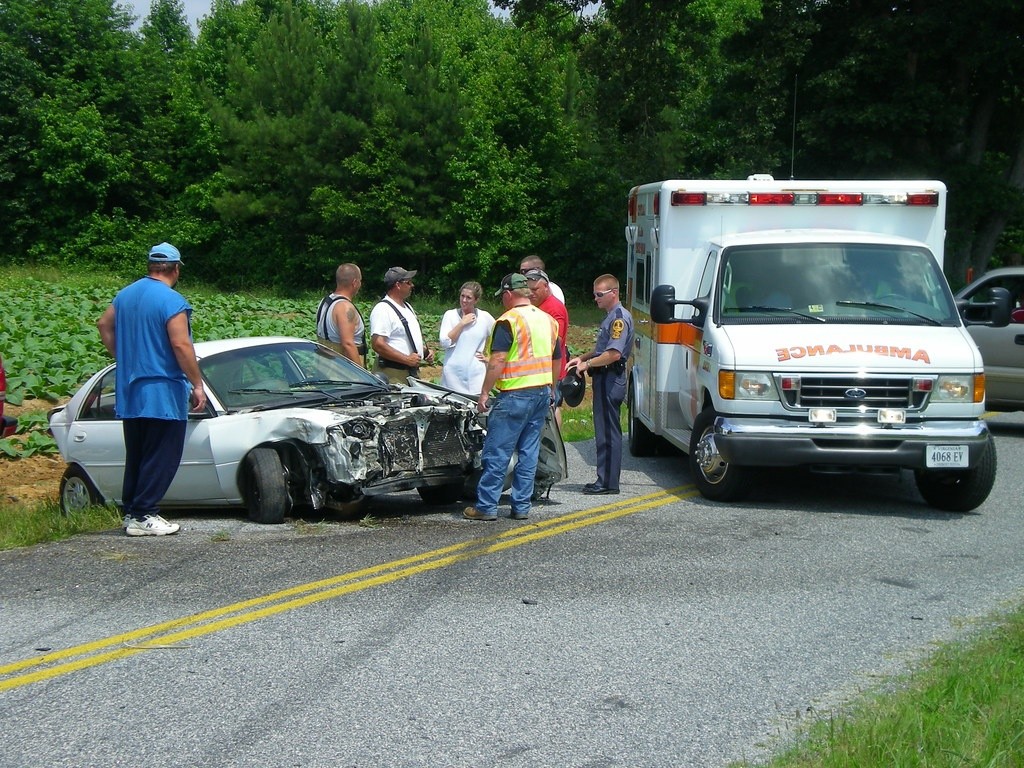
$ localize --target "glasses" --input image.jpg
[592,289,612,300]
[520,266,540,275]
[400,280,413,286]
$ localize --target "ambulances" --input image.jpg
[619,176,998,514]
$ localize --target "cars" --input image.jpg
[44,336,570,526]
[950,267,1024,413]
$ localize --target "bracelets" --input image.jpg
[587,359,592,369]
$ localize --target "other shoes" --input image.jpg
[463,506,498,522]
[510,510,528,521]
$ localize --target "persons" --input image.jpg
[463,273,562,521]
[315,263,368,381]
[520,255,569,430]
[96,242,207,536]
[439,282,496,394]
[370,267,435,386]
[565,274,635,495]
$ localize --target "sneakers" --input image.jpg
[121,514,131,529]
[125,513,180,536]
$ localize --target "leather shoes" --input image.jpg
[582,482,621,495]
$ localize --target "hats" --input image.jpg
[494,272,529,296]
[383,266,418,290]
[148,242,185,267]
[524,269,550,284]
[558,366,587,407]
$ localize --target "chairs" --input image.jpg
[847,278,893,301]
[748,289,793,309]
